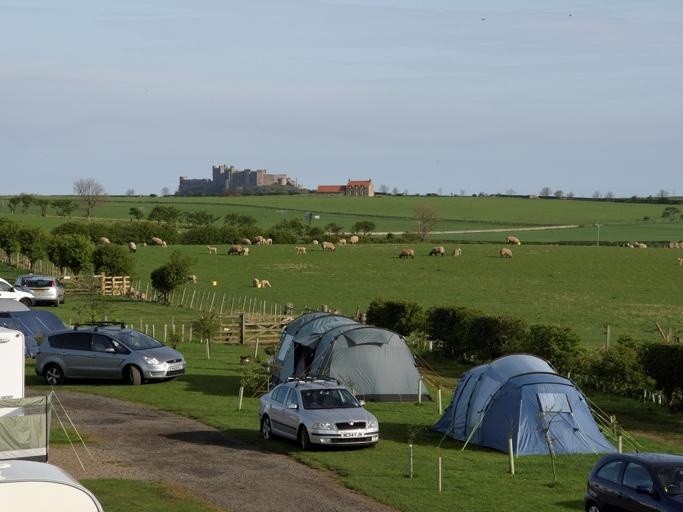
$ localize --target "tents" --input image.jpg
[267,311,435,402]
[432,352,621,456]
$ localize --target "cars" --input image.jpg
[15,276,65,306]
[0,278,36,307]
[260,381,382,452]
[588,454,681,507]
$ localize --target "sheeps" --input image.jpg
[187,274,198,285]
[295,234,360,255]
[634,241,647,248]
[99,236,112,245]
[428,246,446,256]
[399,248,414,259]
[499,236,521,259]
[128,236,167,254]
[253,277,272,289]
[204,235,274,256]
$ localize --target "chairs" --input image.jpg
[307,392,338,408]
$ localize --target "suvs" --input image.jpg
[36,322,187,384]
